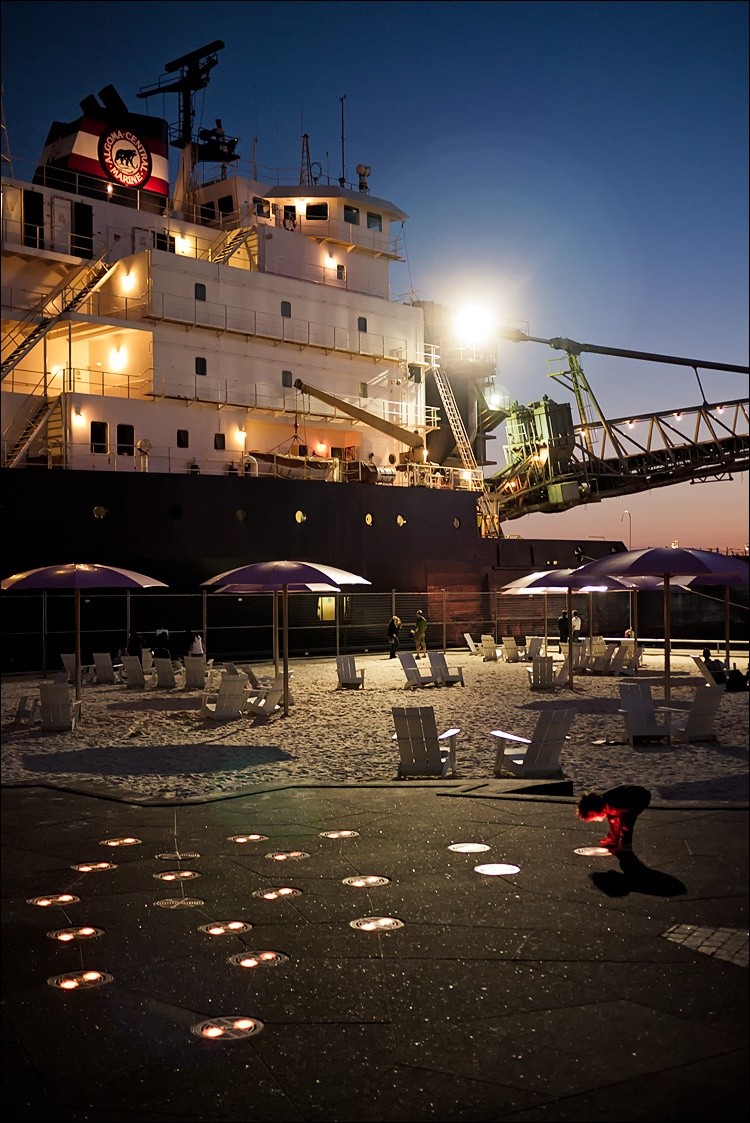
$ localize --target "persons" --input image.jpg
[703,648,728,685]
[157,633,171,658]
[571,610,581,642]
[188,626,205,657]
[575,784,651,856]
[127,629,142,656]
[413,610,427,660]
[387,615,402,659]
[558,610,569,654]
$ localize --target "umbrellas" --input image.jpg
[657,563,750,671]
[620,576,691,675]
[568,546,750,725]
[200,560,371,716]
[0,563,169,723]
[502,589,589,656]
[501,567,640,688]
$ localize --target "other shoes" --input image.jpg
[609,848,634,857]
[599,838,619,848]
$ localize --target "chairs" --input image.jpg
[391,706,460,779]
[464,633,731,777]
[335,655,365,691]
[15,652,293,733]
[397,651,464,690]
